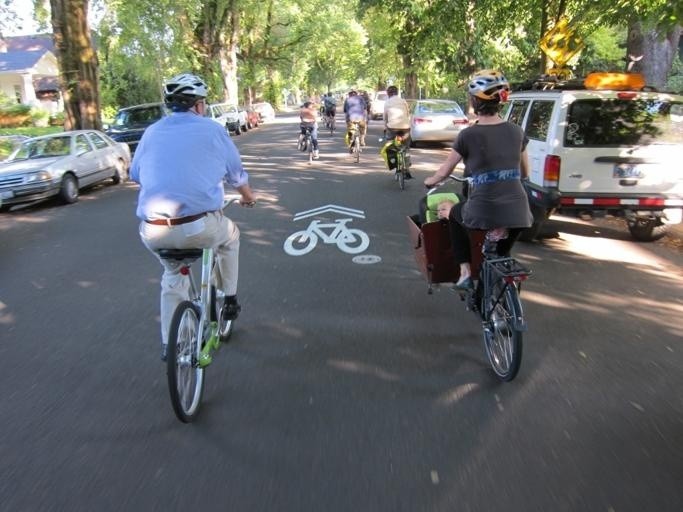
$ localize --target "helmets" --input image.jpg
[164,74,208,110]
[469,70,510,99]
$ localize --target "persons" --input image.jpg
[320,90,373,155]
[421,66,535,297]
[125,71,256,362]
[299,100,320,159]
[380,84,414,178]
[435,199,456,219]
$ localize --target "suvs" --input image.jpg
[500,78,682,242]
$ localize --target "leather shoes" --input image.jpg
[450,277,475,291]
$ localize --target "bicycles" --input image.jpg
[298,104,362,166]
[159,199,254,423]
[424,173,532,382]
[378,126,406,190]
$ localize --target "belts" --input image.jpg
[145,210,216,227]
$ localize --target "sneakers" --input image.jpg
[224,304,241,320]
[161,345,180,360]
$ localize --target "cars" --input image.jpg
[0,128,132,213]
[103,102,171,159]
[403,99,471,148]
[205,101,276,136]
[369,91,389,120]
